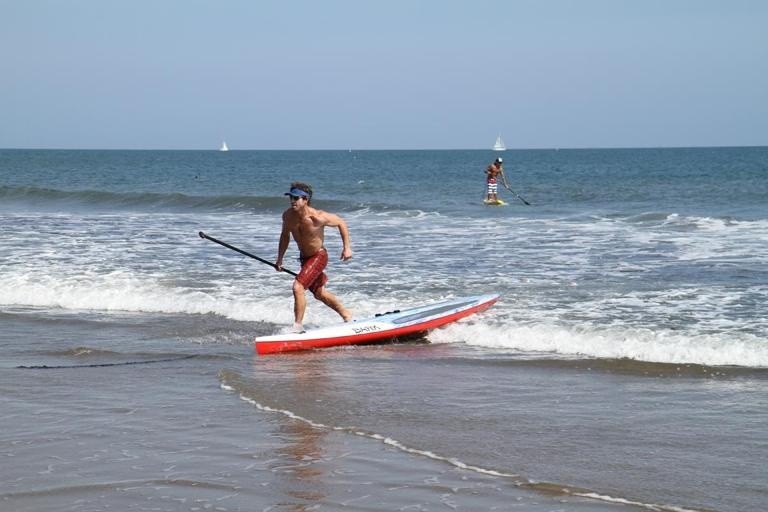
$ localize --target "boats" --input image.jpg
[484,198,504,206]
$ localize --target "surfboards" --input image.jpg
[483,200,504,205]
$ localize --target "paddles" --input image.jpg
[490,176,530,205]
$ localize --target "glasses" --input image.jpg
[289,195,300,200]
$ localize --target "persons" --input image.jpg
[483,156,509,203]
[274,181,356,335]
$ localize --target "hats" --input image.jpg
[284,189,310,198]
[496,158,503,163]
